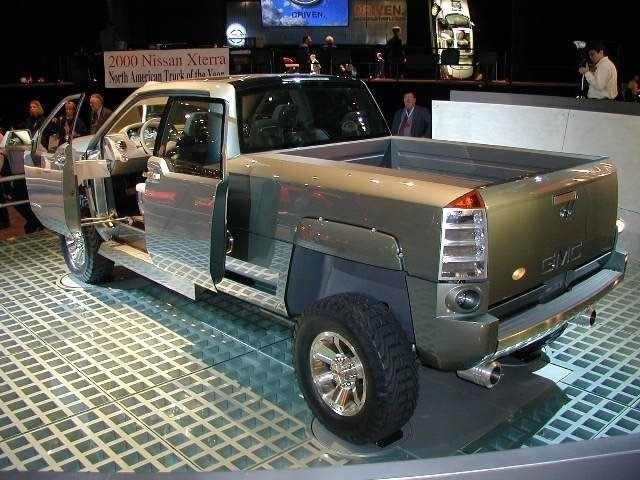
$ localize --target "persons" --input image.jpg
[579,44,618,99]
[391,91,431,138]
[297,26,406,80]
[441,39,460,65]
[625,75,640,99]
[0,94,112,233]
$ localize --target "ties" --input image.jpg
[91,111,98,125]
[397,109,411,136]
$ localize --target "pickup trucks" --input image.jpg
[2,73,629,445]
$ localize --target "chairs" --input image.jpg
[172,104,301,170]
